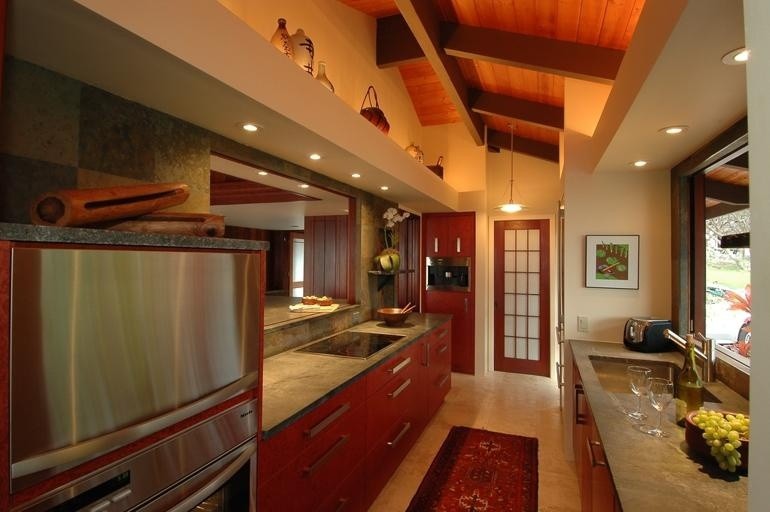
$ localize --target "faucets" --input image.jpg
[662,328,715,383]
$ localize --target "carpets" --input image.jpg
[404,426,539,512]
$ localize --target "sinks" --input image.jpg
[588,354,723,404]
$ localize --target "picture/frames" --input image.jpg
[586,234,640,290]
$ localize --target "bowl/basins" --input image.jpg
[377,307,413,326]
[684,408,748,471]
[301,299,317,305]
[316,299,335,305]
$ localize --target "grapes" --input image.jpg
[692,407,750,474]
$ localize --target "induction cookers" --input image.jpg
[296,330,407,362]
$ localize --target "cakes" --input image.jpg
[303,294,332,306]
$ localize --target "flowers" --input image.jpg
[374,208,411,254]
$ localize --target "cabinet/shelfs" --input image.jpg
[422,211,475,257]
[420,291,474,375]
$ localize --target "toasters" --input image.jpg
[622,315,673,352]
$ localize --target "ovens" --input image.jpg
[425,256,471,292]
[5,246,262,511]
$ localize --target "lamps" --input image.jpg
[494,124,525,213]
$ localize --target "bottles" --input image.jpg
[289,27,314,78]
[271,18,293,62]
[314,61,335,93]
[674,334,703,428]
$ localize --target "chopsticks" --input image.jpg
[401,302,416,314]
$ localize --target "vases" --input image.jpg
[380,255,399,270]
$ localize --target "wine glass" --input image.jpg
[626,366,652,420]
[648,377,675,437]
